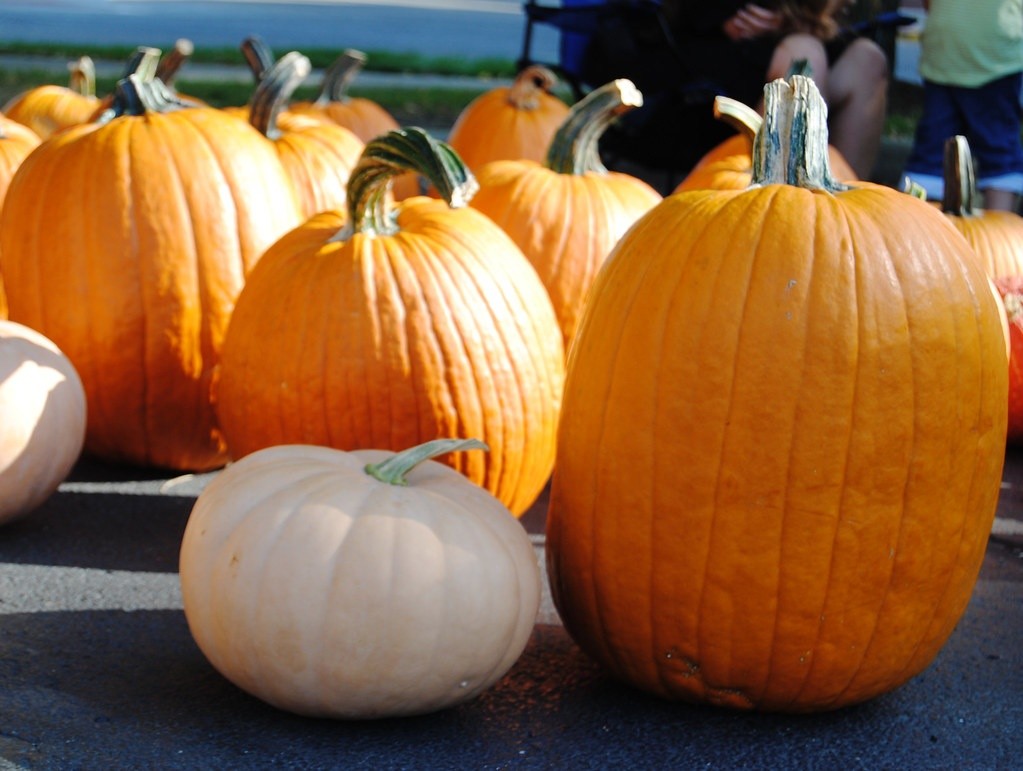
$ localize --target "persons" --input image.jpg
[578,2,900,185]
[904,0,1023,218]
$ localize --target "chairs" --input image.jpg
[516,0,915,192]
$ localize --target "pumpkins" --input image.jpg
[0,34,1023,715]
